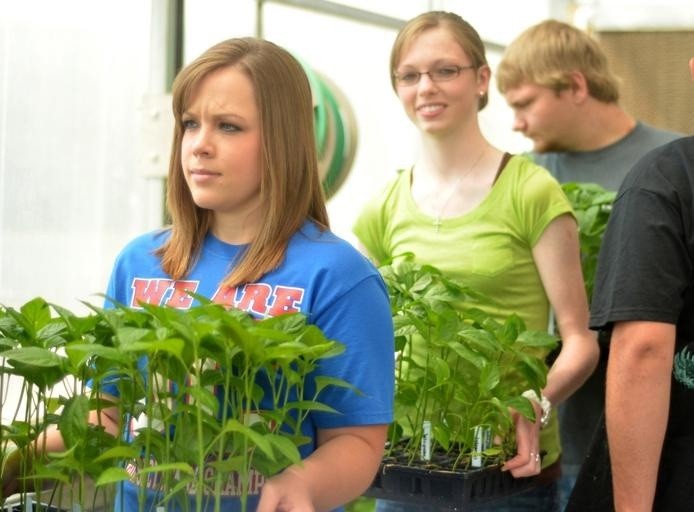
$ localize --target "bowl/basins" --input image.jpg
[424,141,492,235]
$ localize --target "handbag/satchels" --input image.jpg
[519,388,555,432]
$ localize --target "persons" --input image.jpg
[494,16,692,510]
[338,4,602,511]
[561,139,694,511]
[0,35,398,512]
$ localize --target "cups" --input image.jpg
[392,62,477,84]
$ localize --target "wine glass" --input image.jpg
[526,448,542,462]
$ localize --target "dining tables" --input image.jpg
[369,252,557,510]
